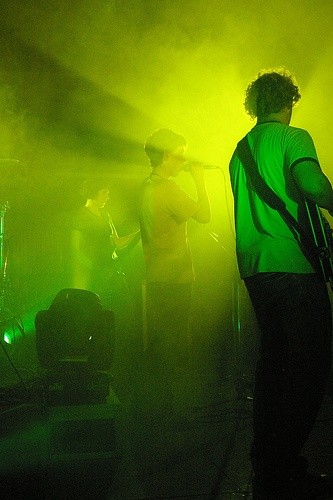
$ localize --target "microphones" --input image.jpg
[183,163,219,172]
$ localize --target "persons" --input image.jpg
[131,127,212,408]
[224,67,333,482]
[76,177,141,385]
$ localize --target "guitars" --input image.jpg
[302,196,332,283]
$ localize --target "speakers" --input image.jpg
[35,287,115,370]
